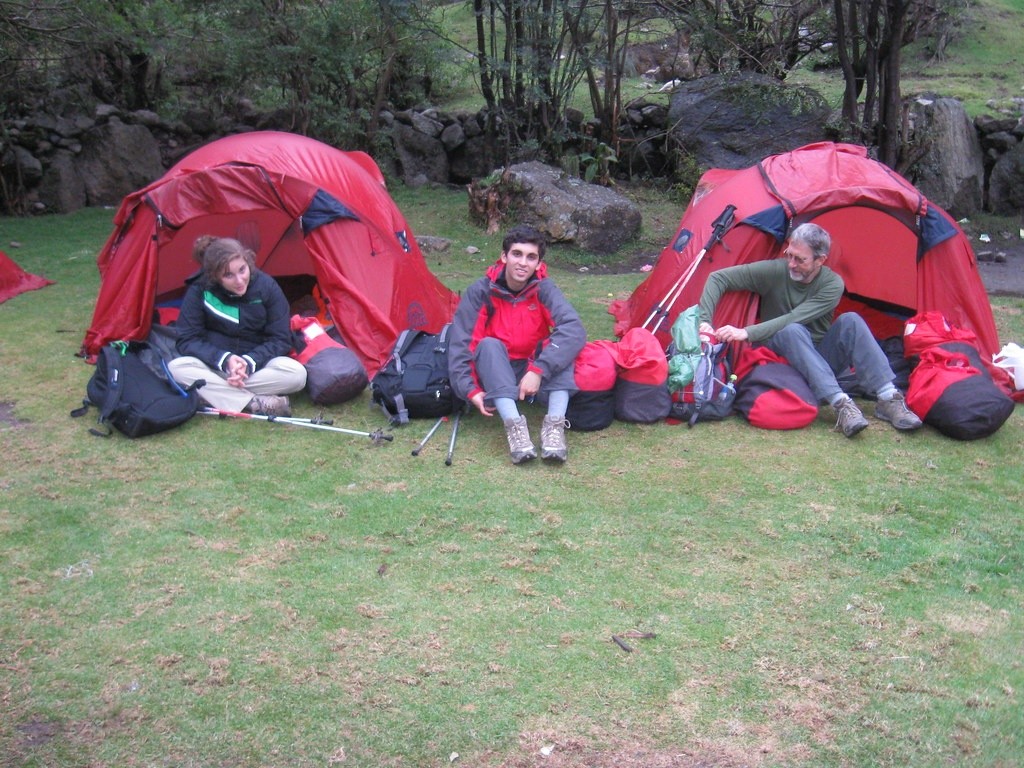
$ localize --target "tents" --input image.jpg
[607,140,1024,425]
[77,130,461,383]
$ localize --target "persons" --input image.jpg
[168,235,307,418]
[696,221,923,437]
[447,226,585,462]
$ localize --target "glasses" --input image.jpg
[782,248,809,264]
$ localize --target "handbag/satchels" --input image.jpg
[903,310,977,358]
[284,314,370,406]
[532,336,617,432]
[905,341,1016,441]
[592,328,674,424]
[732,346,818,429]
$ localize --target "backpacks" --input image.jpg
[70,338,206,440]
[665,332,737,428]
[370,322,466,431]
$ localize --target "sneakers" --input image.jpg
[539,414,571,462]
[831,396,869,439]
[873,393,924,432]
[250,394,292,418]
[503,413,538,466]
